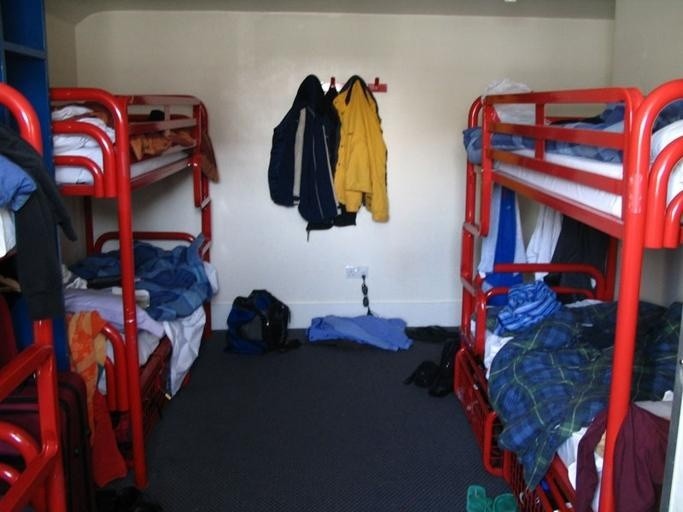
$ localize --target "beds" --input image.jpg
[453,78,683,511]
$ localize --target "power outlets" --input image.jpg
[346,267,367,279]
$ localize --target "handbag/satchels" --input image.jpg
[226,289,288,356]
[497,281,563,334]
